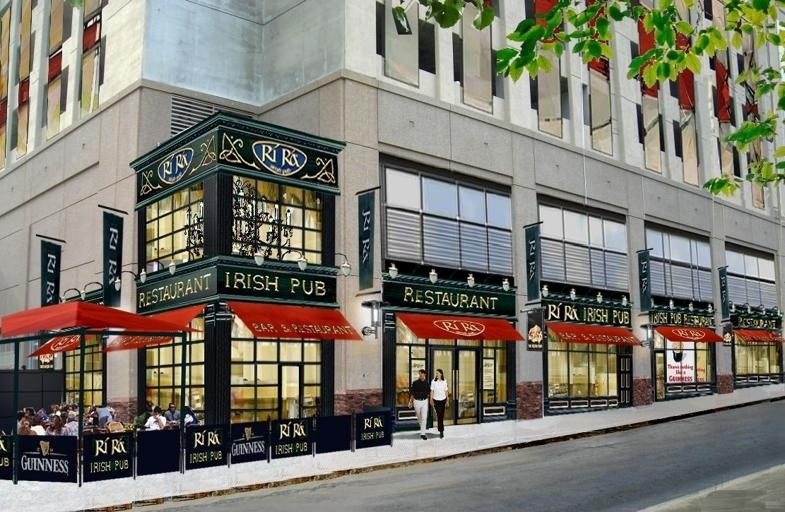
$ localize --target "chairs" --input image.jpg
[104,419,126,433]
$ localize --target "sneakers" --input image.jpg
[421,435,427,440]
[439,430,444,440]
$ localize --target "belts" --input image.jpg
[414,397,429,400]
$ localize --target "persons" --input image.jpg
[429,367,450,439]
[408,368,431,441]
[9,398,202,436]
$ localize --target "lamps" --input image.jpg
[58,247,195,305]
[238,243,352,277]
[541,285,629,307]
[386,260,510,292]
[360,299,389,339]
[639,322,660,347]
